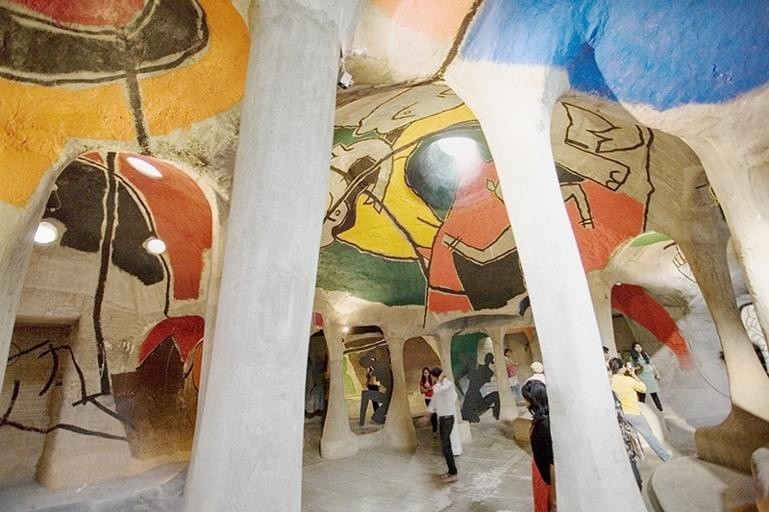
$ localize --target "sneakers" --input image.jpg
[441,473,457,483]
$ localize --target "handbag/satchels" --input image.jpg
[506,364,519,377]
[624,421,646,462]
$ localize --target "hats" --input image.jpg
[529,362,543,374]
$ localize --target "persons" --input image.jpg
[420,367,438,437]
[419,368,458,483]
[720,343,769,381]
[523,343,672,510]
[366,358,380,412]
[505,348,526,406]
